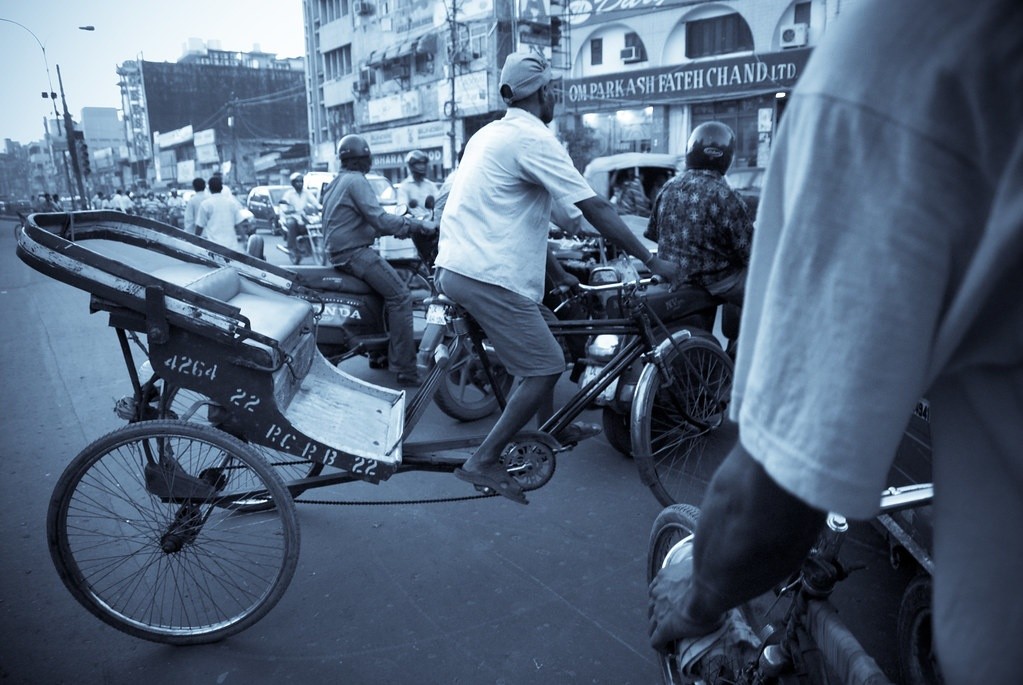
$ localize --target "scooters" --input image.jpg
[579,254,739,458]
[248,196,440,367]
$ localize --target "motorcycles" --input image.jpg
[416,229,604,420]
[275,199,327,267]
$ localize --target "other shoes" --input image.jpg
[368,352,389,368]
[397,372,424,386]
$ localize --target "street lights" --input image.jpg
[0,19,95,211]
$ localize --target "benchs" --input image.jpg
[181,267,312,368]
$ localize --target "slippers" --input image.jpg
[454,460,530,505]
[561,421,603,446]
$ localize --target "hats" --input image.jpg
[498,52,562,107]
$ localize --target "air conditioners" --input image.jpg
[778,23,807,48]
[353,80,367,93]
[353,1,370,15]
[618,46,642,62]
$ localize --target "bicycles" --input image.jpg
[645,482,934,685]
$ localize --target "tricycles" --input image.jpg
[15,210,736,645]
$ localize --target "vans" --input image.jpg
[303,172,399,213]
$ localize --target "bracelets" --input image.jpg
[644,254,653,265]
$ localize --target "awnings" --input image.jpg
[364,33,437,66]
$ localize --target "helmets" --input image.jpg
[340,137,371,161]
[405,150,430,164]
[289,172,303,182]
[686,121,737,176]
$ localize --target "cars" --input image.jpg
[184,192,256,237]
[3,195,30,212]
[726,167,766,216]
[248,185,293,235]
[380,182,442,202]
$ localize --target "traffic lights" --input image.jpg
[77,143,91,175]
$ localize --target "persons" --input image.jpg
[184,172,248,253]
[281,172,323,258]
[320,137,440,385]
[647,0,1023,685]
[31,188,187,228]
[643,122,754,365]
[610,169,672,216]
[432,52,684,505]
[396,150,440,218]
